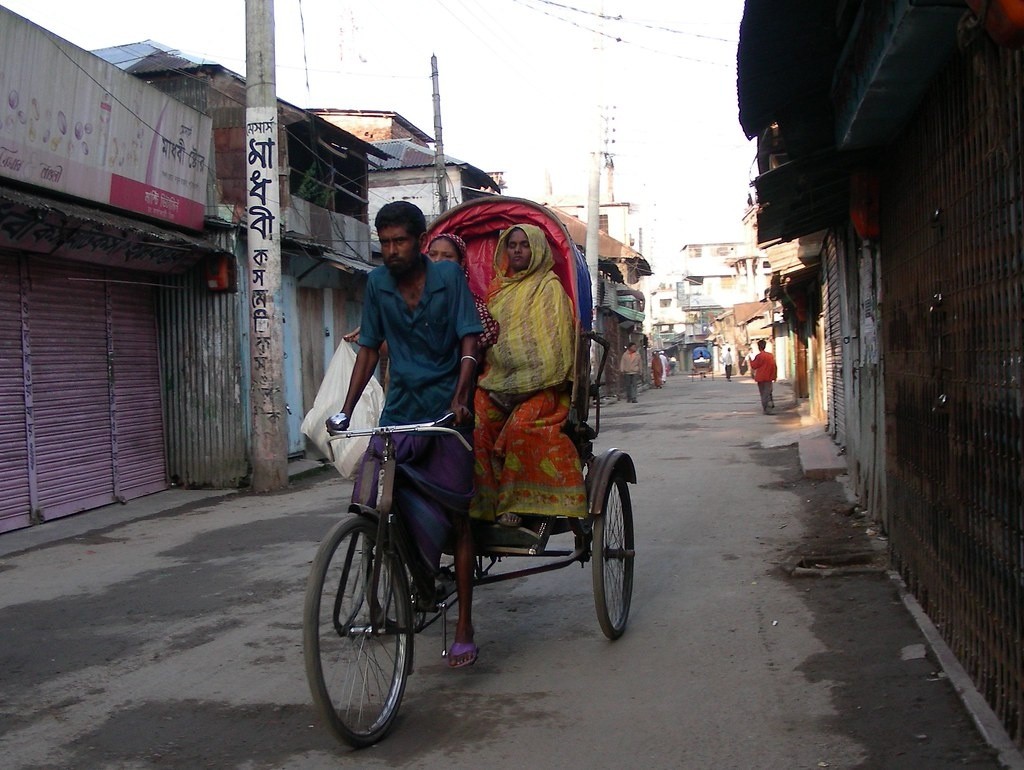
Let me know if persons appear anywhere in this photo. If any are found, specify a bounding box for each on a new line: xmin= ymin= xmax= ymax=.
xmin=343 ymin=234 xmax=500 ymax=349
xmin=670 ymin=355 xmax=676 ymax=361
xmin=659 ymin=351 xmax=668 ymax=382
xmin=651 ymin=352 xmax=663 ymax=388
xmin=749 ymin=340 xmax=777 ymax=415
xmin=724 ymin=347 xmax=732 ymax=379
xmin=326 ymin=201 xmax=483 ymax=668
xmin=698 ymin=351 xmax=706 ymax=377
xmin=471 ymin=224 xmax=588 ymax=525
xmin=742 ymin=348 xmax=756 ymax=379
xmin=621 ymin=342 xmax=642 ymax=403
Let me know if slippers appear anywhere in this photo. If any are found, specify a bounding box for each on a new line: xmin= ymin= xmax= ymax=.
xmin=447 ymin=642 xmax=476 ymax=669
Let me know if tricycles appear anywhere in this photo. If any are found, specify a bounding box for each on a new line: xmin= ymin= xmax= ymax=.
xmin=691 ymin=347 xmax=714 ymax=383
xmin=302 ymin=195 xmax=637 ymax=748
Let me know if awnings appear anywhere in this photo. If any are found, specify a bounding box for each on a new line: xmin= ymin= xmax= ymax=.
xmin=612 ymin=305 xmax=645 ymax=322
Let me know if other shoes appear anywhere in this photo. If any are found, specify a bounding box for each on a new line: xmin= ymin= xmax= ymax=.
xmin=498 ymin=513 xmax=522 ymax=527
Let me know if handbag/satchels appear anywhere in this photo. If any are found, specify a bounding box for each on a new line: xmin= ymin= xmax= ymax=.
xmin=299 ymin=338 xmax=386 ymax=483
